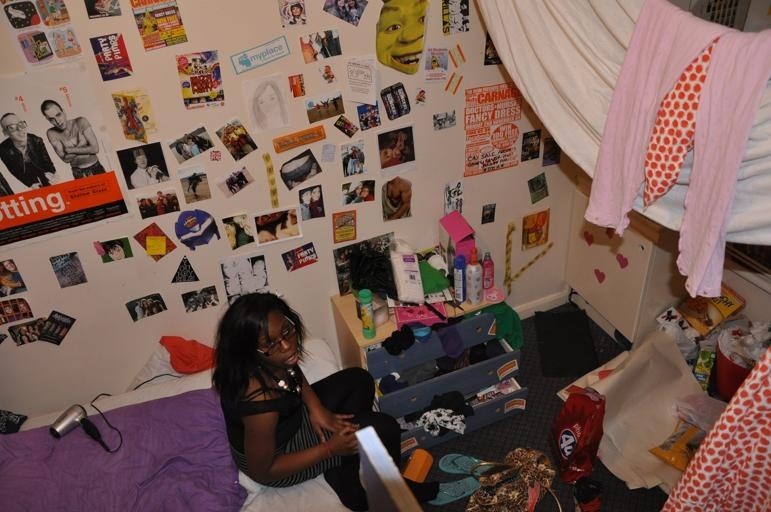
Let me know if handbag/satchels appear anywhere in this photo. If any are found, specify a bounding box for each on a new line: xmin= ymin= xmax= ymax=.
xmin=554 ymin=391 xmax=606 ymax=485
xmin=463 ymin=445 xmax=557 ymax=512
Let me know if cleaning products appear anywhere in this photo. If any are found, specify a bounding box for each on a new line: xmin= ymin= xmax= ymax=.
xmin=483 ymin=252 xmax=494 ymax=289
xmin=465 ymin=248 xmax=483 ymax=305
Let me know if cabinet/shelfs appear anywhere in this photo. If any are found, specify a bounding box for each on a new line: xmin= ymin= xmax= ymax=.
xmin=567 ymin=187 xmax=690 ymax=351
xmin=330 ymin=297 xmax=530 ymax=460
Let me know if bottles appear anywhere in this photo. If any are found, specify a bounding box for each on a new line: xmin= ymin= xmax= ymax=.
xmin=358 ymin=287 xmax=377 ymax=340
xmin=452 ymin=248 xmax=495 ymax=305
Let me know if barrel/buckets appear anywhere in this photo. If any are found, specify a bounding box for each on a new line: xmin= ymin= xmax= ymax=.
xmin=712 ymin=337 xmax=751 ymax=401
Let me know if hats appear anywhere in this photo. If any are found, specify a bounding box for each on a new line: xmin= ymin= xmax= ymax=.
xmin=347 ymin=180 xmax=363 ymax=194
xmin=133 ymin=148 xmax=146 ymax=159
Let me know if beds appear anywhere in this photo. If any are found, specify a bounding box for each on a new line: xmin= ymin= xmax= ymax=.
xmin=1 ymin=338 xmax=367 ymax=512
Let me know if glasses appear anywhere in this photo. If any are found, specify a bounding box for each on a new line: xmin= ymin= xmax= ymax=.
xmin=255 ymin=315 xmax=297 ymax=358
xmin=4 ymin=120 xmax=28 ymax=133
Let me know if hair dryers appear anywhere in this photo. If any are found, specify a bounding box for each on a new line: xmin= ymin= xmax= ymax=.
xmin=49 ymin=405 xmax=112 ymax=455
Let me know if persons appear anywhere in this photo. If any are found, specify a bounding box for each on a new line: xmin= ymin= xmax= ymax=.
xmin=0 ymin=259 xmax=29 ymax=296
xmin=40 ymin=99 xmax=107 ymax=180
xmin=133 ymin=297 xmax=165 ymax=320
xmin=341 ymin=145 xmax=365 ymax=177
xmin=135 ymin=190 xmax=179 ymax=220
xmin=220 ymin=257 xmax=267 ymax=305
xmin=102 ymin=238 xmax=126 ymax=261
xmin=173 ymin=132 xmax=212 ymax=160
xmin=361 ymin=186 xmax=376 ymax=202
xmin=224 ymin=170 xmax=248 ymax=194
xmin=342 ymin=181 xmax=365 ymax=207
xmin=446 ymin=180 xmax=464 ymax=214
xmin=229 ymin=133 xmax=258 ymax=162
xmin=117 ymin=96 xmax=138 ymax=129
xmin=311 ymin=97 xmax=339 ymax=121
xmin=207 ymin=291 xmax=439 ymax=512
xmin=375 ymin=0 xmax=428 ymax=75
xmin=415 ymin=89 xmax=427 ymax=102
xmin=331 ymin=0 xmax=353 ymax=22
xmin=310 ymin=186 xmax=326 ymax=219
xmin=187 ymin=172 xmax=201 ymax=199
xmin=430 ymin=55 xmax=441 ymax=71
xmin=286 ymin=3 xmax=308 ymax=28
xmin=232 ymin=214 xmax=254 ymax=247
xmin=322 ymin=65 xmax=336 ymax=85
xmin=1 ymin=301 xmax=68 ymax=346
xmin=185 ymin=291 xmax=217 ymax=314
xmin=222 ymin=223 xmax=237 ymax=249
xmin=251 ymin=78 xmax=289 ymax=128
xmin=381 ymin=176 xmax=413 ymax=222
xmin=129 ymin=148 xmax=164 ymax=187
xmin=346 ymin=0 xmax=363 ymax=21
xmin=0 ymin=113 xmax=59 ymax=193
xmin=299 ymin=189 xmax=313 ymax=222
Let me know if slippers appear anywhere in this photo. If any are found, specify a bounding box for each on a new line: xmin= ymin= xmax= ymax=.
xmin=427 ymin=477 xmax=482 ymax=507
xmin=438 ymin=453 xmax=495 ymax=476
